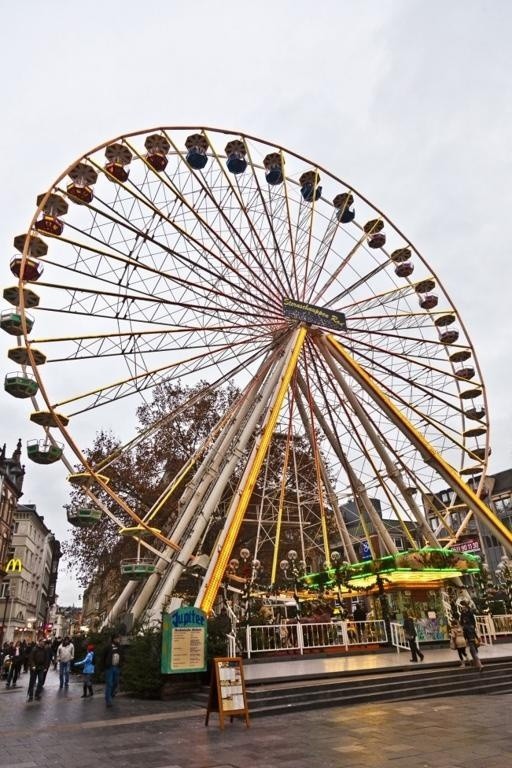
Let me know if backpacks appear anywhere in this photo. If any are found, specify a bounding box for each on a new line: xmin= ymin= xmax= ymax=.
xmin=92 ymin=657 xmax=96 ymax=664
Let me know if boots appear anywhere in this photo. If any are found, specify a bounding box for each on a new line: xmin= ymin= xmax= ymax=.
xmin=475 ymin=659 xmax=484 ymax=672
xmin=466 ymin=657 xmax=471 ymax=662
xmin=460 ymin=661 xmax=465 ymax=667
xmin=81 ymin=685 xmax=93 ymax=698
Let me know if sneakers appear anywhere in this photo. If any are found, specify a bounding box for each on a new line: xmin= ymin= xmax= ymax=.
xmin=27 ymin=696 xmax=41 ymax=702
xmin=420 ymin=654 xmax=425 ymax=661
xmin=410 ymin=658 xmax=418 ymax=662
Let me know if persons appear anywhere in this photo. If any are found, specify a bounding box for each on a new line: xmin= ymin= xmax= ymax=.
xmin=101 ymin=633 xmax=123 ymax=707
xmin=401 ymin=611 xmax=425 ymax=662
xmin=462 ymin=615 xmax=484 ymax=672
xmin=449 ymin=619 xmax=472 ymax=669
xmin=74 ymin=644 xmax=96 ymax=699
xmin=460 ymin=600 xmax=477 ymax=630
xmin=26 ymin=638 xmax=52 ymax=702
xmin=352 ymin=604 xmax=365 ymax=635
xmin=0 ymin=635 xmax=76 ymax=691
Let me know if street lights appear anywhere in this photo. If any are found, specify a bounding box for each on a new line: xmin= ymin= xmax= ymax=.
xmin=0 ymin=589 xmax=10 ymax=630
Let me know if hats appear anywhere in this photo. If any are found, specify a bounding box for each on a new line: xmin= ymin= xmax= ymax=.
xmin=87 ymin=644 xmax=95 ymax=651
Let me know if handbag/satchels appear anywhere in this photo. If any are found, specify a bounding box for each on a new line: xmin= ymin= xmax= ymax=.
xmin=405 ymin=633 xmax=414 ymax=641
xmin=449 ymin=639 xmax=456 ymax=650
xmin=474 ymin=637 xmax=482 ymax=648
